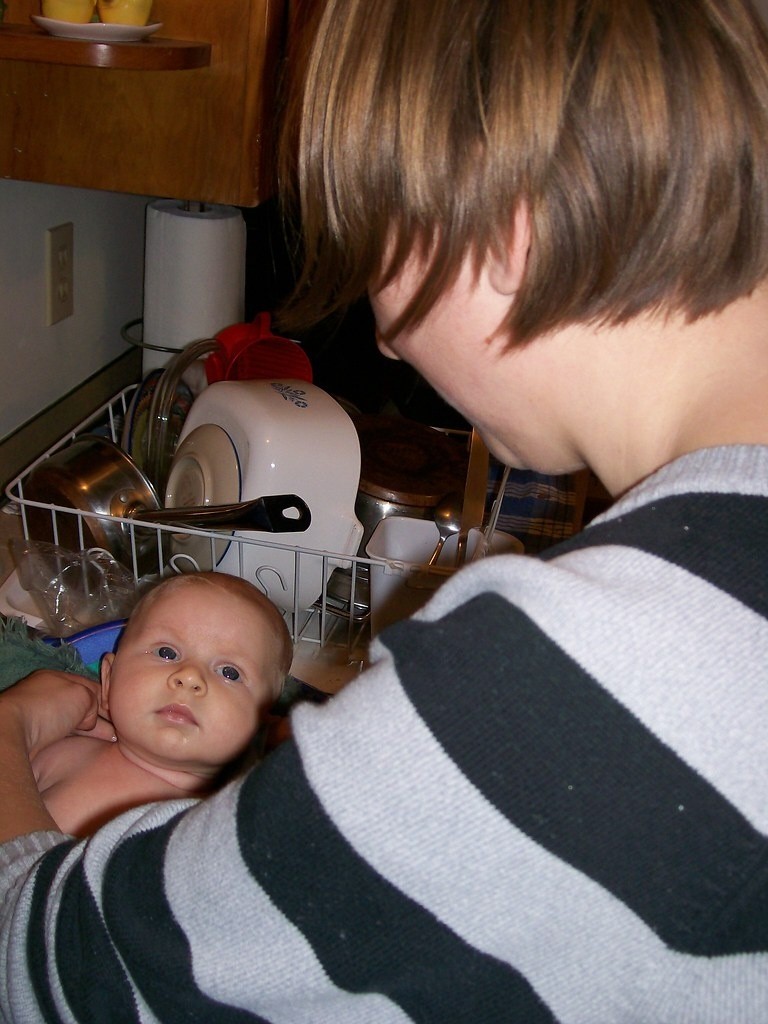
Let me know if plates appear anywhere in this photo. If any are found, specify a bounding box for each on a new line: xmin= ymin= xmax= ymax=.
xmin=120 ymin=338 xmax=218 ymax=506
xmin=162 ymin=423 xmax=242 ymax=574
xmin=30 ymin=14 xmax=164 ymax=41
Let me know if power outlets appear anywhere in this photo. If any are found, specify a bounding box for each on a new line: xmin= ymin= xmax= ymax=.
xmin=46 ymin=222 xmax=73 ymax=328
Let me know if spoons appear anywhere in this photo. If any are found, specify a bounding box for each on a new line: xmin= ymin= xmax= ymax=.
xmin=427 ymin=495 xmax=462 ymax=563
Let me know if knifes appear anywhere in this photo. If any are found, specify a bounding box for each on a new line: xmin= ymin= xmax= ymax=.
xmin=458 ymin=426 xmax=490 ymax=567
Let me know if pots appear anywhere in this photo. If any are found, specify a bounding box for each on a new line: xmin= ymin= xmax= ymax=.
xmin=24 ymin=433 xmax=312 ymax=592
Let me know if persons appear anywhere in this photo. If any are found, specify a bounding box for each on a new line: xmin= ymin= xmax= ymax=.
xmin=0 ymin=1 xmax=767 ymax=1024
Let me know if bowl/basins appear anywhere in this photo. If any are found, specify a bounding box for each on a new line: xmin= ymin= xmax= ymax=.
xmin=175 ymin=378 xmax=364 ymax=614
